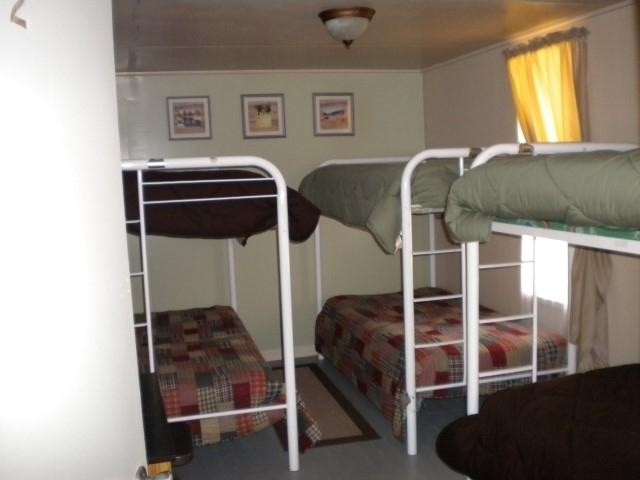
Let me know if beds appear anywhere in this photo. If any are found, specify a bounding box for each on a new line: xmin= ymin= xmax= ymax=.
xmin=436 ymin=137 xmax=640 ymax=476
xmin=121 ymin=155 xmax=323 ymax=471
xmin=298 ymin=147 xmax=542 ymax=455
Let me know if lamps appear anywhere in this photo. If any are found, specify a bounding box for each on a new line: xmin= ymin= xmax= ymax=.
xmin=317 ymin=6 xmax=377 ymax=48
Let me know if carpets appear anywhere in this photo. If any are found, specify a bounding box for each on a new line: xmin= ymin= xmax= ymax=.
xmin=270 ymin=362 xmax=382 ymax=452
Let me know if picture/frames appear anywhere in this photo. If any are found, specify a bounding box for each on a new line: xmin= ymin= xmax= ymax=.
xmin=312 ymin=91 xmax=355 ymax=137
xmin=166 ymin=93 xmax=212 ymax=141
xmin=241 ymin=92 xmax=286 ymax=140
